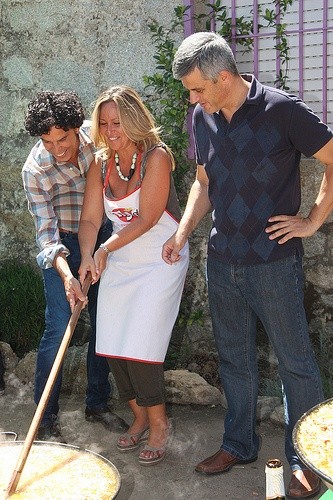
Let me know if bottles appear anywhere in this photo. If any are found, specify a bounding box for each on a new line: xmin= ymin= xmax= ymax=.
xmin=265 ymin=458 xmax=286 ymax=500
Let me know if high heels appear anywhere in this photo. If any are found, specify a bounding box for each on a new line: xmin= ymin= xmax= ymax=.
xmin=117 ymin=423 xmax=149 ymax=451
xmin=139 ymin=419 xmax=174 ymax=466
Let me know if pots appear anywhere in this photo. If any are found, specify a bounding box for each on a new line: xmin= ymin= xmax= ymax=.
xmin=0 ymin=431 xmax=122 ymax=500
xmin=292 ymin=396 xmax=333 ymax=492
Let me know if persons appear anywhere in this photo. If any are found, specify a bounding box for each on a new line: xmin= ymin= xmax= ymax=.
xmin=22 ymin=92 xmax=130 ymax=443
xmin=162 ymin=32 xmax=333 ymax=500
xmin=77 ymin=84 xmax=190 ymax=464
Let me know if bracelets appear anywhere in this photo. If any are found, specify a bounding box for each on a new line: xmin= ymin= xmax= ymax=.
xmin=100 ymin=244 xmax=111 ymax=255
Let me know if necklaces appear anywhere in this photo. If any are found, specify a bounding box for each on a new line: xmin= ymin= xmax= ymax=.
xmin=115 ymin=151 xmax=136 ymax=182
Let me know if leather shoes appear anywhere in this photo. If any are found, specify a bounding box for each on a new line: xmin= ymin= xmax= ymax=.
xmin=285 ymin=469 xmax=322 ymax=500
xmin=36 ymin=423 xmax=66 ymax=443
xmin=87 ymin=408 xmax=130 ymax=434
xmin=195 ymin=448 xmax=258 ymax=475
